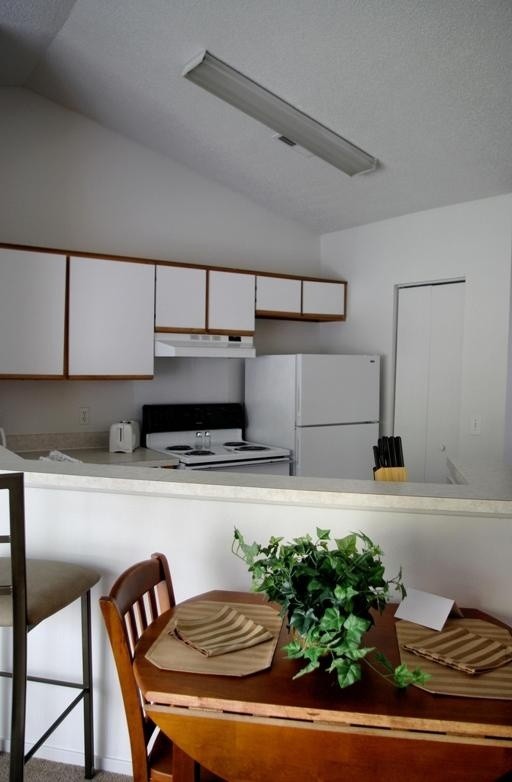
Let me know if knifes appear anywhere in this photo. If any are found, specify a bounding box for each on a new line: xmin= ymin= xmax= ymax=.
xmin=372 ymin=436 xmax=405 ymax=468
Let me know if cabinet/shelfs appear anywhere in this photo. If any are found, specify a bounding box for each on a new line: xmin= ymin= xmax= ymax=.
xmin=2 ymin=242 xmax=348 ymax=381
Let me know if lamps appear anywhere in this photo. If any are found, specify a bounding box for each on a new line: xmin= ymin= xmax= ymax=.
xmin=181 ymin=49 xmax=378 ymax=180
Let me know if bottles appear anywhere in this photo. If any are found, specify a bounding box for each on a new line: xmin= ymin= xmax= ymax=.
xmin=195 ymin=431 xmax=211 ymax=450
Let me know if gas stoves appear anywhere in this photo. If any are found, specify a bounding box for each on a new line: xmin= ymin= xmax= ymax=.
xmin=142 ymin=440 xmax=291 ymax=465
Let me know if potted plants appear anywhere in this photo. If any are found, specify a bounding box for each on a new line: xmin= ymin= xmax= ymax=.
xmin=229 ymin=524 xmax=433 ymax=690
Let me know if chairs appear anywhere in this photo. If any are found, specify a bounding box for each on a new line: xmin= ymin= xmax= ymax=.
xmin=98 ymin=551 xmax=224 ymax=780
xmin=0 ymin=471 xmax=107 ymax=780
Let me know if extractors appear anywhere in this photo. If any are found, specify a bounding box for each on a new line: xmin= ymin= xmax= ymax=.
xmin=154 ymin=332 xmax=257 ymax=359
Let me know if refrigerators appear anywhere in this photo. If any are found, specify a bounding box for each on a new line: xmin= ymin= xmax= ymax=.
xmin=244 ymin=353 xmax=380 ymax=481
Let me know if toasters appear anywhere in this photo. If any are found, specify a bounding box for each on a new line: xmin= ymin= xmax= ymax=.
xmin=109 ymin=420 xmax=140 ymax=454
